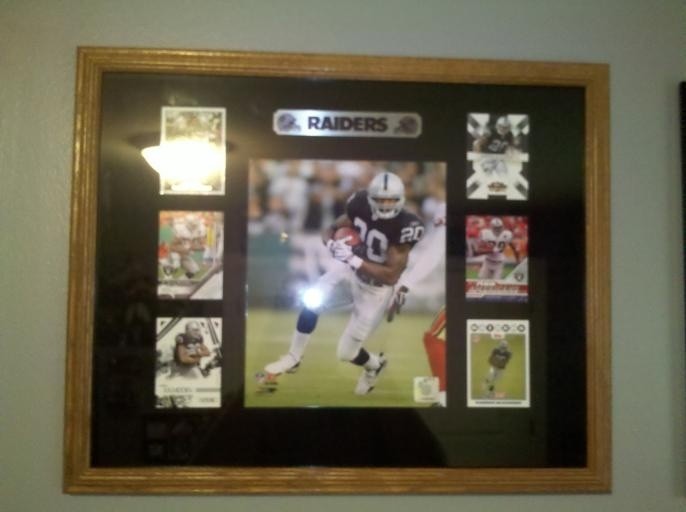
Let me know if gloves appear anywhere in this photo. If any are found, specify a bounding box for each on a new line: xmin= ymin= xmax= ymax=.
xmin=326 ymin=235 xmax=364 ymax=272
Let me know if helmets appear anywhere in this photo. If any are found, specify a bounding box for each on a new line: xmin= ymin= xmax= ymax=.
xmin=366 ymin=171 xmax=407 ymax=220
xmin=490 ymin=218 xmax=504 ymax=236
xmin=496 ymin=117 xmax=510 ymax=136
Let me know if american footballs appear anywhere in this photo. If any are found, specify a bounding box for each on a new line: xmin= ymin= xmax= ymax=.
xmin=334 ymin=226 xmax=361 ymax=247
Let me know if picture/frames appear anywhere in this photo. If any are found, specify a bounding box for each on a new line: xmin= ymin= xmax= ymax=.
xmin=62 ymin=42 xmax=617 ymax=501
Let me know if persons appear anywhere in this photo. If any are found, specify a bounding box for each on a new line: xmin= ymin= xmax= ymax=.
xmin=473 ymin=118 xmax=516 ymax=196
xmin=486 ymin=340 xmax=512 ymax=391
xmin=164 ymin=216 xmax=208 ymax=279
xmin=264 ymin=172 xmax=426 ymax=396
xmin=474 ymin=217 xmax=513 ymax=279
xmin=170 ymin=320 xmax=210 ymax=380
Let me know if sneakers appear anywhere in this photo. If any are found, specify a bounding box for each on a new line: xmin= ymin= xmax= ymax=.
xmin=355 ymin=354 xmax=385 ymax=394
xmin=264 ymin=353 xmax=300 ymax=375
